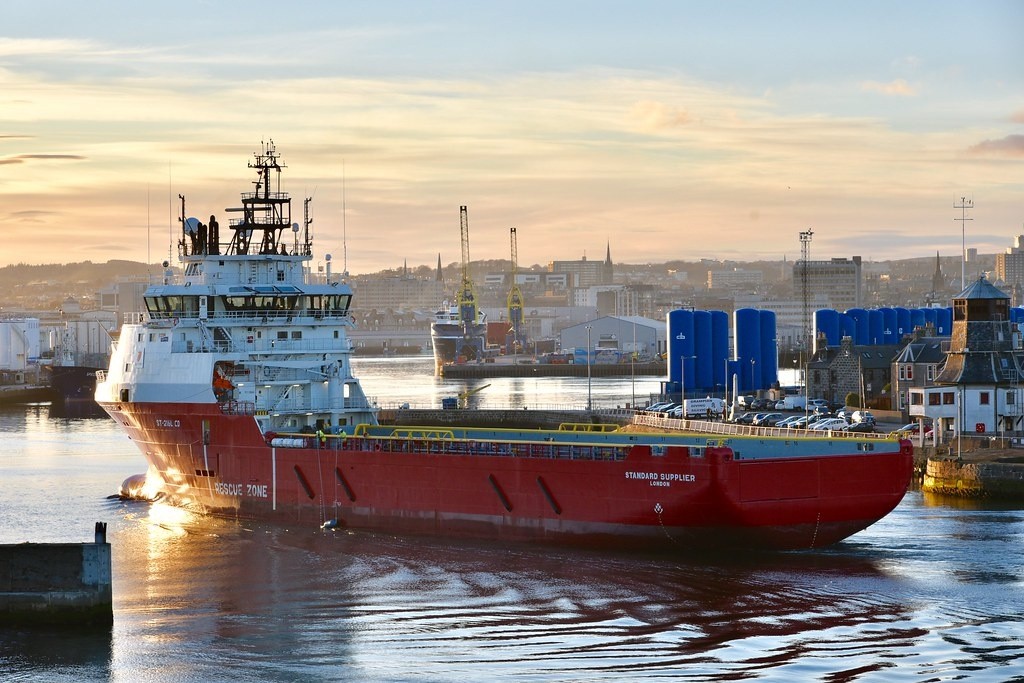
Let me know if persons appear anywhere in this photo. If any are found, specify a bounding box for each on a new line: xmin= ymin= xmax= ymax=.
xmin=715 ymin=409 xmax=718 ymax=422
xmin=316 ymin=430 xmax=326 ymax=449
xmin=722 ymin=406 xmax=726 ymax=420
xmin=337 ymin=428 xmax=348 ymax=450
xmin=707 ymin=407 xmax=712 ymax=422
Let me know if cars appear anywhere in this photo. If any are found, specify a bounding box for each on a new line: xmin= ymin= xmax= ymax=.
xmin=644 ymin=396 xmax=877 ymax=433
xmin=897 ymin=423 xmax=932 ymax=436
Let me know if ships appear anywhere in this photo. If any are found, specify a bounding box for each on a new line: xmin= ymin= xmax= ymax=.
xmin=89 ymin=132 xmax=916 ymax=574
